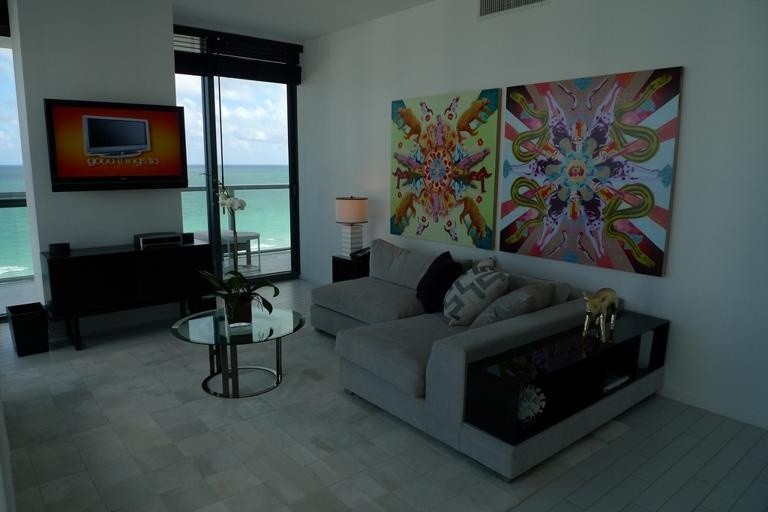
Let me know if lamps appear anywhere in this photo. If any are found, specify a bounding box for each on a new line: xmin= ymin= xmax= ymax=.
xmin=336 ymin=195 xmax=368 ymax=257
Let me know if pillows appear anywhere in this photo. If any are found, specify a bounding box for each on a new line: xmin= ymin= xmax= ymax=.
xmin=415 ymin=250 xmax=555 ymax=327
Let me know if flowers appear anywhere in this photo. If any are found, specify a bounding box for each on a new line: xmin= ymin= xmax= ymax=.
xmin=197 ymin=172 xmax=280 ymax=315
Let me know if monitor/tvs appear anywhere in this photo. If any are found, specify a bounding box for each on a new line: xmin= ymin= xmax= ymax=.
xmin=82 ymin=114 xmax=151 ymax=159
xmin=43 ymin=97 xmax=189 ymax=192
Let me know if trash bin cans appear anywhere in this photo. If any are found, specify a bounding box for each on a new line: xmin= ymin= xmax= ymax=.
xmin=6 ymin=302 xmax=48 ymax=357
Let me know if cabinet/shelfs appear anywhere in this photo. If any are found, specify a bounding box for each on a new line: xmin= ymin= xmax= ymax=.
xmin=40 ymin=238 xmax=214 ymax=351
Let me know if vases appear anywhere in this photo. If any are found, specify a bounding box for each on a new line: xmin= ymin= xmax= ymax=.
xmin=228 ymin=299 xmax=251 ymax=324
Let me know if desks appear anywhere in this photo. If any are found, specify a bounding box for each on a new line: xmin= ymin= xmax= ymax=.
xmin=333 ymin=255 xmax=369 ymax=283
xmin=194 ymin=232 xmax=261 ymax=276
